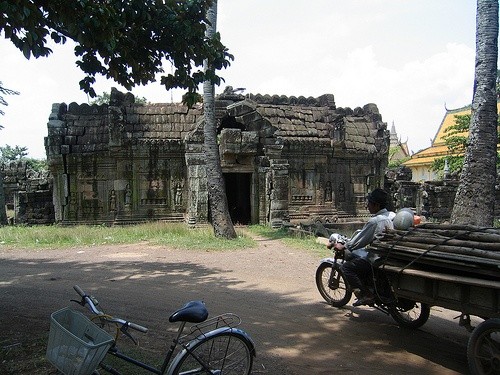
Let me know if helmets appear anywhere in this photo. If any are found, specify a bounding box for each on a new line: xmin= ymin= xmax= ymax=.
xmin=393 ymin=208 xmax=414 ymax=229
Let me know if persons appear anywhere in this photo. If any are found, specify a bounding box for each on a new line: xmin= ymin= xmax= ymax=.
xmin=125 ymin=184 xmax=131 ymax=205
xmin=109 ymin=189 xmax=117 ymax=211
xmin=333 ymin=188 xmax=397 ymax=306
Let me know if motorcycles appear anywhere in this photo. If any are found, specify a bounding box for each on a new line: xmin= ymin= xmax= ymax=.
xmin=316 ymin=222 xmax=500 ymax=375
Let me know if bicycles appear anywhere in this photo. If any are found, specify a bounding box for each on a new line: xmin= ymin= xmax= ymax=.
xmin=44 ymin=284 xmax=257 ymax=375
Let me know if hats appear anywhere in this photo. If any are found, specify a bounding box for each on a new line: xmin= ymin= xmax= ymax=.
xmin=365 ymin=188 xmax=391 ymax=206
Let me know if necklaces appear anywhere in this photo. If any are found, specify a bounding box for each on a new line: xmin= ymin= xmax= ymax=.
xmin=175 ymin=182 xmax=181 ymax=205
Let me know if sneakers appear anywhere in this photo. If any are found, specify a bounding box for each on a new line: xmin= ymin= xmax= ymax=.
xmin=353 ymin=296 xmax=375 ymax=307
